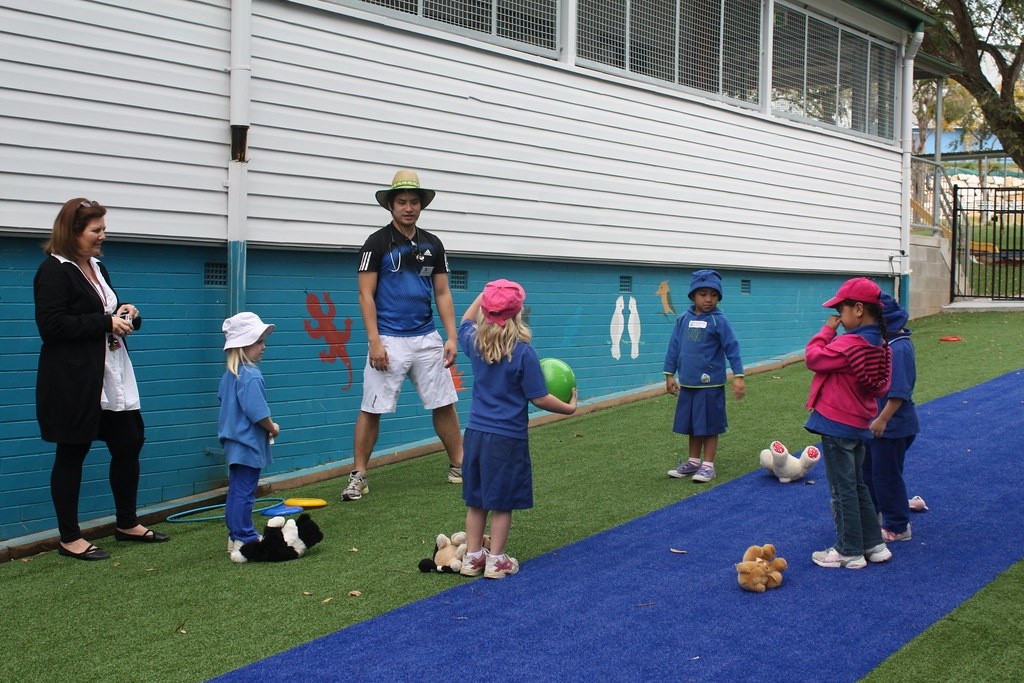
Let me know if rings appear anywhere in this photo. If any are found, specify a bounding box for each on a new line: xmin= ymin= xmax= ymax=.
xmin=119 ymin=330 xmax=124 ymax=335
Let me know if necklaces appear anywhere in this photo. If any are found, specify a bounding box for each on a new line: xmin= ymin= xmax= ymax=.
xmin=76 ymin=261 xmax=121 ymax=351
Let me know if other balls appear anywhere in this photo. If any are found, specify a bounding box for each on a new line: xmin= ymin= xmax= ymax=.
xmin=539 ymin=358 xmax=576 ymax=403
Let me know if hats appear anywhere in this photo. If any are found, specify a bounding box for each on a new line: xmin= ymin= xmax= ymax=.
xmin=374 ymin=170 xmax=436 ymax=211
xmin=222 ymin=311 xmax=275 ymax=351
xmin=688 ymin=270 xmax=722 ymax=302
xmin=822 ymin=277 xmax=884 ymax=308
xmin=479 ymin=279 xmax=526 ymax=326
xmin=881 ymin=294 xmax=909 ymax=333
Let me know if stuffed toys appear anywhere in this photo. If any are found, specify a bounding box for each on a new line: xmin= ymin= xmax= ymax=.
xmin=239 ymin=513 xmax=324 ymax=563
xmin=418 ymin=531 xmax=493 ymax=574
xmin=759 ymin=441 xmax=820 ymax=484
xmin=908 ymin=496 xmax=928 ymax=511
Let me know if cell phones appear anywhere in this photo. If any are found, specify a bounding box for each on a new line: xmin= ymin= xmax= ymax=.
xmin=119 ymin=315 xmax=141 ymax=330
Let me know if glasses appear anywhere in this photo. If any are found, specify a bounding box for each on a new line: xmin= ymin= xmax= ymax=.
xmin=77 ymin=201 xmax=99 ymax=211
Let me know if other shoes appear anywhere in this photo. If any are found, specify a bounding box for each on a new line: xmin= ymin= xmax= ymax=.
xmin=115 ymin=529 xmax=169 ymax=542
xmin=59 ymin=542 xmax=110 ymax=561
xmin=228 ymin=534 xmax=263 ymax=555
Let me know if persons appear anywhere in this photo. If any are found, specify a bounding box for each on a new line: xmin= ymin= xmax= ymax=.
xmin=663 ymin=270 xmax=746 ymax=482
xmin=805 ymin=277 xmax=892 ymax=569
xmin=458 ymin=279 xmax=577 ymax=579
xmin=871 ymin=294 xmax=920 ymax=542
xmin=217 ymin=312 xmax=279 ymax=553
xmin=341 ymin=171 xmax=466 ymax=501
xmin=33 ymin=198 xmax=169 ymax=561
xmin=736 ymin=544 xmax=788 ymax=592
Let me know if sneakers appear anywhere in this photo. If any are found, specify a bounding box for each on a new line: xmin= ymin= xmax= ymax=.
xmin=881 ymin=523 xmax=912 ymax=542
xmin=877 ymin=512 xmax=882 ymax=526
xmin=448 ymin=464 xmax=463 ymax=483
xmin=811 ymin=547 xmax=867 ymax=569
xmin=341 ymin=470 xmax=369 ymax=501
xmin=484 ymin=554 xmax=519 ymax=579
xmin=863 ymin=543 xmax=892 ymax=562
xmin=691 ymin=466 xmax=716 ymax=483
xmin=667 ymin=460 xmax=701 ymax=478
xmin=460 ymin=547 xmax=490 ymax=576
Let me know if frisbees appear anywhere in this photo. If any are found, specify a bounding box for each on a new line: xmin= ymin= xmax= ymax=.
xmin=261 ymin=505 xmax=304 ymax=516
xmin=284 ymin=498 xmax=327 ymax=507
